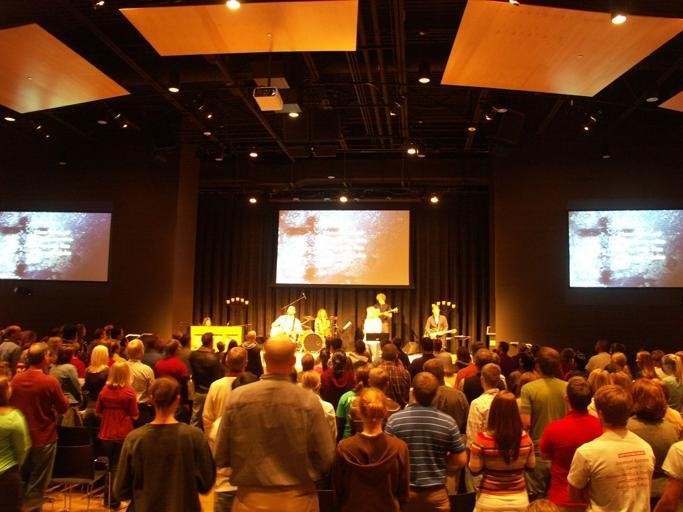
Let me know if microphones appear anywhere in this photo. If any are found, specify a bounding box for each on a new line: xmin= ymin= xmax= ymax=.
xmin=303 ymin=292 xmax=307 ymax=299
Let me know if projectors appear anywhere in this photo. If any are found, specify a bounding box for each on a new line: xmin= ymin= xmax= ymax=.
xmin=253 ymin=87 xmax=283 ymax=111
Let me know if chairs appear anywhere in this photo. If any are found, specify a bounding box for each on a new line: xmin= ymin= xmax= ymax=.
xmin=51 ymin=426 xmax=111 ymax=512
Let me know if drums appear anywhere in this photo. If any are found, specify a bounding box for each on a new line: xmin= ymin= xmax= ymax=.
xmin=302 ymin=333 xmax=324 ymax=352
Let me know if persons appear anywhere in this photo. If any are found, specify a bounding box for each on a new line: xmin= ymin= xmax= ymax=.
xmin=202 ymin=293 xmax=448 ymax=347
xmin=0 ymin=324 xmax=682 ymax=512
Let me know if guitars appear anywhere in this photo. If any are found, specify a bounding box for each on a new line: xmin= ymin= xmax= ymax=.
xmin=422 ymin=329 xmax=457 ymax=341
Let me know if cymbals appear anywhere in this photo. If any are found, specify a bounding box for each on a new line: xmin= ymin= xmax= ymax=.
xmin=301 ymin=316 xmax=314 ymax=320
xmin=326 ymin=316 xmax=339 ymax=320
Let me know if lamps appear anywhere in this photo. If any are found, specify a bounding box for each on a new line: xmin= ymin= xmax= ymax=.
xmin=611 ymin=8 xmax=627 ymax=25
xmin=418 ymin=62 xmax=431 ymax=83
